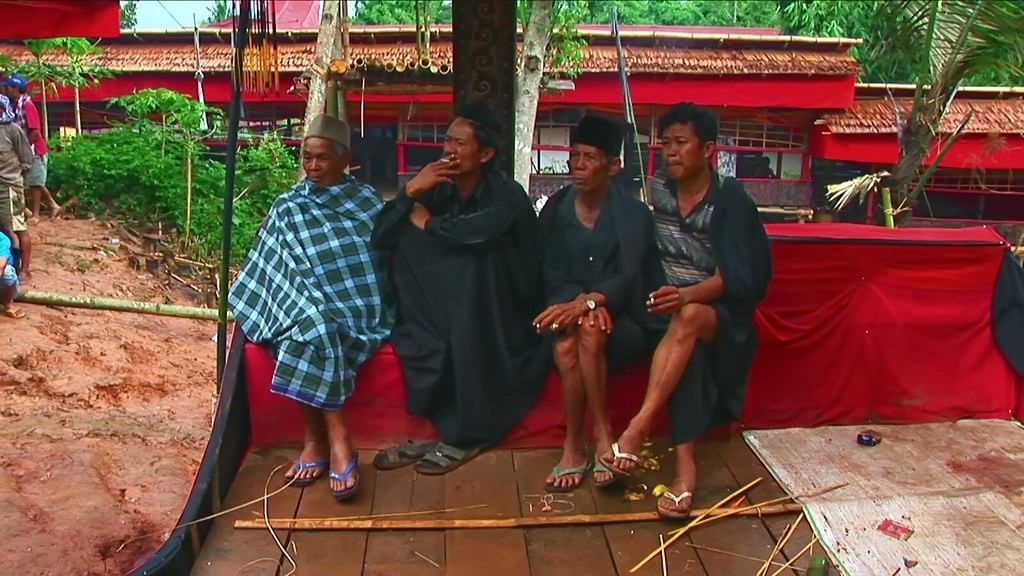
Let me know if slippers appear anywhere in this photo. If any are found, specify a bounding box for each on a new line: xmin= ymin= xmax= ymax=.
xmin=598 ymin=442 xmax=643 ymax=477
xmin=543 ymin=455 xmax=592 ymax=493
xmin=285 ymin=460 xmax=326 ymax=483
xmin=328 ymin=451 xmax=360 ymax=499
xmin=592 ymin=467 xmax=619 ymax=487
xmin=656 ymin=490 xmax=693 ymax=519
xmin=413 ymin=441 xmax=481 ymax=474
xmin=372 ymin=439 xmax=439 ymax=469
xmin=5 ymin=307 xmax=25 ymax=317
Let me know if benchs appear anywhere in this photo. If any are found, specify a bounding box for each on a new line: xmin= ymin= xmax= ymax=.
xmin=247 ymin=341 xmax=742 ymax=455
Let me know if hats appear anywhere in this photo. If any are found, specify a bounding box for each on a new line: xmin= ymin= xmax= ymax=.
xmin=305 ymin=114 xmax=349 ymax=151
xmin=571 ymin=112 xmax=627 ymax=156
xmin=0 ymin=73 xmax=26 ymax=88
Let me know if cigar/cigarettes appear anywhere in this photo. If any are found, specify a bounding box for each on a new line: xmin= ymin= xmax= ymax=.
xmin=441 ymin=159 xmax=450 ymax=163
xmin=651 ymin=298 xmax=654 ymax=304
xmin=537 ymin=323 xmax=540 ymax=328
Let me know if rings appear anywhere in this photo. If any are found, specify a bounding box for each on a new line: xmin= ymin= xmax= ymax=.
xmin=552 ymin=323 xmax=559 ymax=328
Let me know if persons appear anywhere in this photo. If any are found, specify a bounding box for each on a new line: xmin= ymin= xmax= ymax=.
xmin=371 ymin=110 xmax=537 ymax=447
xmin=0 ymin=73 xmax=61 ymax=317
xmin=532 ymin=113 xmax=652 ymax=492
xmin=227 ymin=114 xmax=390 ymax=499
xmin=600 ymin=101 xmax=772 ymax=519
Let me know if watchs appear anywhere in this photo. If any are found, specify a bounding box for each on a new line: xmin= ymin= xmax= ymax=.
xmin=583 ymin=295 xmax=596 ymax=310
